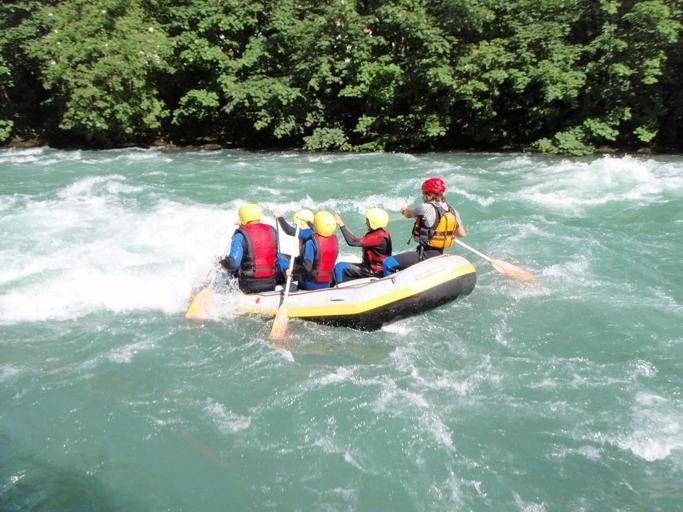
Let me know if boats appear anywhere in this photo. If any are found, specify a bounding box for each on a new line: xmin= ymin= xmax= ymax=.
xmin=184 ymin=247 xmax=476 ymax=332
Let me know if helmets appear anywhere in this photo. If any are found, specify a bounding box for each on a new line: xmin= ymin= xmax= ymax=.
xmin=238 ymin=203 xmax=261 ymax=225
xmin=364 ymin=207 xmax=389 ymax=231
xmin=313 ymin=210 xmax=337 ymax=238
xmin=421 ymin=178 xmax=446 ymax=193
xmin=292 ymin=209 xmax=315 ymax=229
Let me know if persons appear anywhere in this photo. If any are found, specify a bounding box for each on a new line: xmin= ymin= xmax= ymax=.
xmin=223 ymin=203 xmax=280 ymax=292
xmin=331 ymin=208 xmax=391 ymax=286
xmin=281 ymin=211 xmax=339 ymax=293
xmin=391 ymin=177 xmax=465 ymax=270
xmin=270 ymin=206 xmax=314 ymax=282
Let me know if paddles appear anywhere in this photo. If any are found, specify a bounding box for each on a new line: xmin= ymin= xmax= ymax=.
xmin=414 ymin=216 xmax=521 ymax=274
xmin=269 ymin=218 xmax=302 ymax=332
xmin=185 ymin=222 xmax=240 ymax=316
